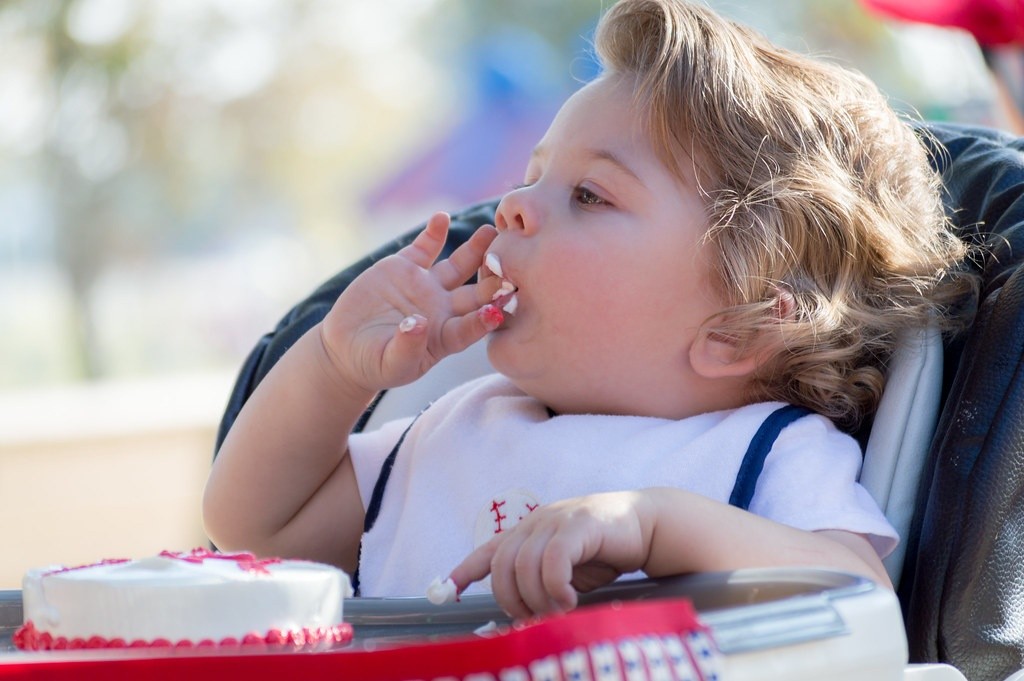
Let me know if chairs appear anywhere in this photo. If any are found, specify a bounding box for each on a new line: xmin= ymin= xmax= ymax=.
xmin=1 ymin=111 xmax=1024 ymax=681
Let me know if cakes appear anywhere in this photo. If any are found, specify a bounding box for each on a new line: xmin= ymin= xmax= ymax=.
xmin=12 ymin=547 xmax=355 ymax=653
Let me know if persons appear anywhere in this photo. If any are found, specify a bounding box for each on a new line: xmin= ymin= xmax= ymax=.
xmin=200 ymin=0 xmax=978 ymax=619
